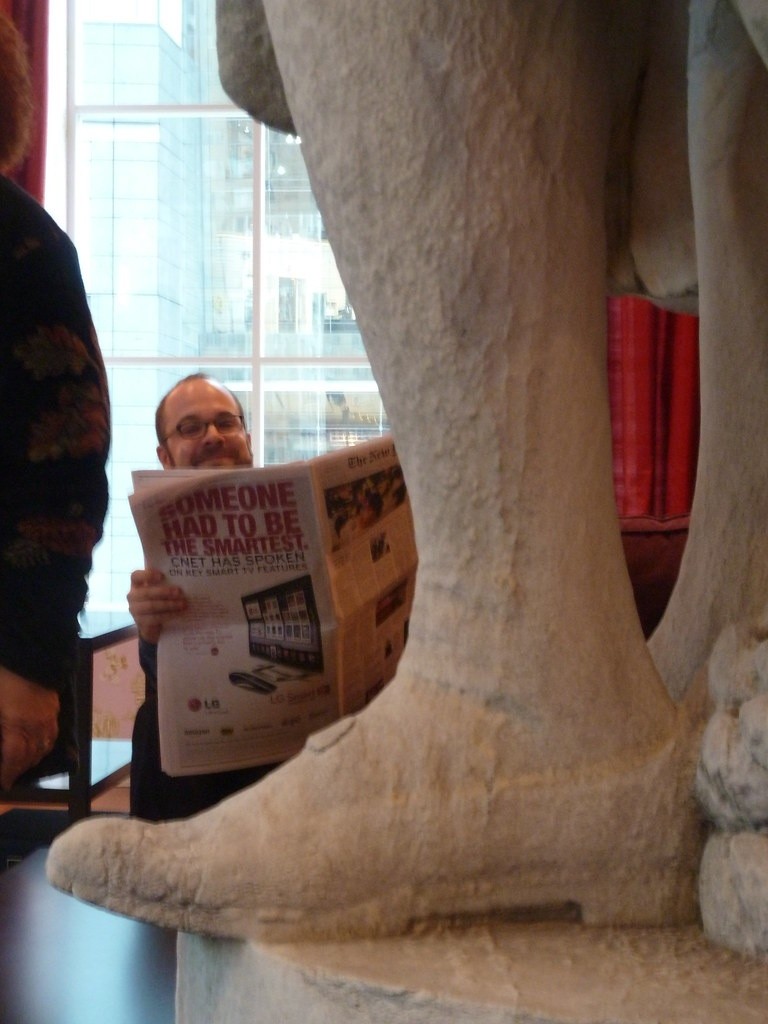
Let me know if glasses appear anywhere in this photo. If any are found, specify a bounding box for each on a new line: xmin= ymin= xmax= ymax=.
xmin=163 ymin=414 xmax=244 ymax=444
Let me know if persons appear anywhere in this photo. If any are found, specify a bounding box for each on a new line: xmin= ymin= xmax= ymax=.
xmin=0 ymin=7 xmax=112 ymax=873
xmin=52 ymin=0 xmax=767 ymax=946
xmin=131 ymin=372 xmax=286 ymax=826
xmin=328 ymin=465 xmax=407 ymax=547
